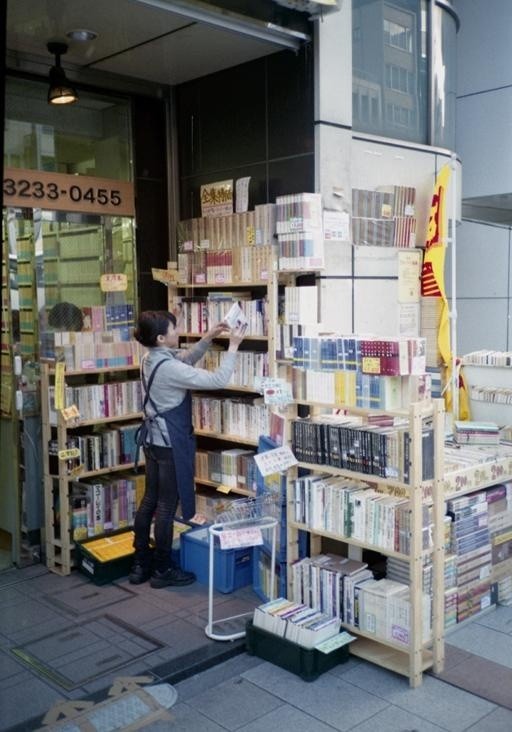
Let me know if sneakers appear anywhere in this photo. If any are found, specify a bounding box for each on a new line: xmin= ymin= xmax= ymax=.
xmin=151 ymin=560 xmax=196 ymax=588
xmin=128 ymin=561 xmax=152 ymax=584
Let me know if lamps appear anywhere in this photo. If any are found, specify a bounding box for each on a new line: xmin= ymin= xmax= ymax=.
xmin=45 ymin=42 xmax=79 ymax=105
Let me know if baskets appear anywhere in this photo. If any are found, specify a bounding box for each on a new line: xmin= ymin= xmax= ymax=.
xmin=216 ymin=493 xmax=276 ymax=530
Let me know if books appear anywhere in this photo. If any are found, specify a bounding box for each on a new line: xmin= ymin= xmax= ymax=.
xmin=42 ymin=209 xmax=138 ymax=306
xmin=42 ymin=306 xmax=157 ymax=563
xmin=173 ymin=193 xmax=511 ymax=651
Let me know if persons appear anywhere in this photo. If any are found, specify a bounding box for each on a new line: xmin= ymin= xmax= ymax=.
xmin=47 ymin=302 xmax=100 ymax=555
xmin=128 ymin=312 xmax=248 ymax=588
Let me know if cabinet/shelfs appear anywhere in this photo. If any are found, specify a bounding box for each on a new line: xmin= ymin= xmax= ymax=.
xmin=0 ymin=217 xmax=512 ymax=690
